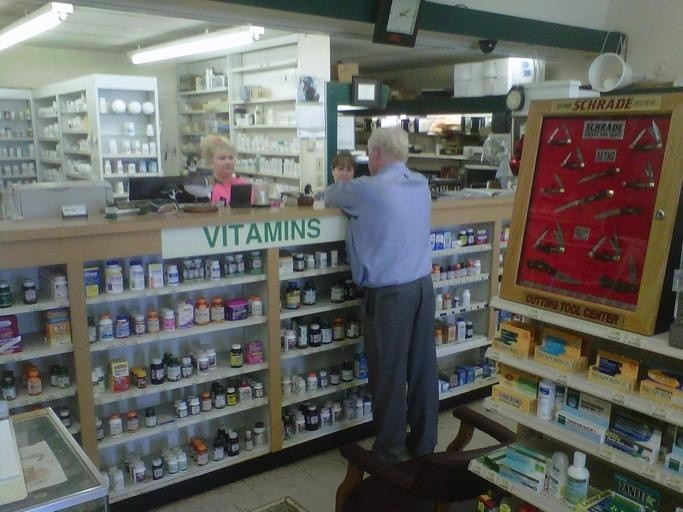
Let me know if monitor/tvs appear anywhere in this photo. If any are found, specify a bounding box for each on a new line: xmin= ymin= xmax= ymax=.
xmin=129 ymin=175 xmax=192 ymax=202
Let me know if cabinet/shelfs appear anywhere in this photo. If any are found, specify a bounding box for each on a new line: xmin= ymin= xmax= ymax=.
xmin=0 ymin=31 xmax=681 ymax=511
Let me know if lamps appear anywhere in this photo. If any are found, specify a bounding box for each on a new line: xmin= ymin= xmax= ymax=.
xmin=1 ymin=2 xmax=73 ymax=53
xmin=127 ymin=25 xmax=265 ymax=66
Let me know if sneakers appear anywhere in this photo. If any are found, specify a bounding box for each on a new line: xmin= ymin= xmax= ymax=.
xmin=405 ymin=431 xmax=435 ymax=458
xmin=371 ymin=439 xmax=412 ymax=463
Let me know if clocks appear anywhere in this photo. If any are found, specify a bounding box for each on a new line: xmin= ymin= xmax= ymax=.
xmin=372 ymin=0 xmax=426 ymax=47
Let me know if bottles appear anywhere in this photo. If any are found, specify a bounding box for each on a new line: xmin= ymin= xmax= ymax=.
xmin=279 ymin=248 xmax=380 ymax=436
xmin=547 ymin=450 xmax=590 ymax=507
xmin=430 ymin=222 xmax=493 ymax=346
xmin=534 ymin=375 xmax=566 ymax=423
xmin=116 ymin=180 xmax=125 ymax=195
xmin=0 ymin=250 xmax=269 ymax=496
xmin=105 ymin=139 xmax=157 ymax=176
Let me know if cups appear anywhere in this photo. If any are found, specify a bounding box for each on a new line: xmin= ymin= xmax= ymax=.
xmin=588 ymin=51 xmax=633 ymax=93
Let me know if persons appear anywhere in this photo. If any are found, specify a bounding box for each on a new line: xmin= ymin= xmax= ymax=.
xmin=193 ymin=133 xmax=253 ymax=206
xmin=331 ymin=150 xmax=357 ymax=184
xmin=325 ymin=126 xmax=438 ymax=491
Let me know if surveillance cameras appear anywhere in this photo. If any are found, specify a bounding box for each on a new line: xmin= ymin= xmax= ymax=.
xmin=479 ymin=40 xmax=496 ymax=53
